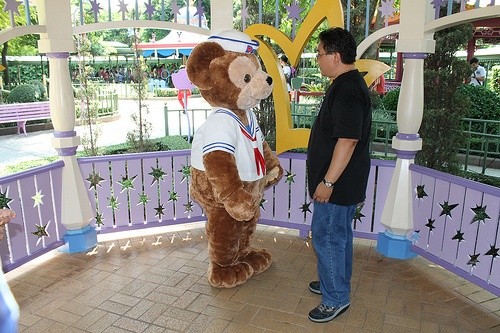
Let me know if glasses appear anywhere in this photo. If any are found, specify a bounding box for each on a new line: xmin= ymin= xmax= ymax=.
xmin=314 ymin=49 xmax=338 ymax=60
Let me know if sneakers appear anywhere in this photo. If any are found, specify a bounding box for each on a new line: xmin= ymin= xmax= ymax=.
xmin=308 ymin=302 xmax=350 ymax=323
xmin=309 ymin=282 xmax=323 ymax=294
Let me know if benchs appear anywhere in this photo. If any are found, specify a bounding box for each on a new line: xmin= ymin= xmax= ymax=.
xmin=0 ymin=101 xmax=50 ymax=137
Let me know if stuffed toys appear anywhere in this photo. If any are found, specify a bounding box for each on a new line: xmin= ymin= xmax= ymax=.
xmin=171 ymin=67 xmax=194 ymax=113
xmin=187 ymin=30 xmax=282 ymax=290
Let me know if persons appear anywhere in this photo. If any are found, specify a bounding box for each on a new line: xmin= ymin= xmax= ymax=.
xmin=0 ymin=208 xmax=19 ymax=333
xmin=69 ymin=58 xmax=184 ymax=89
xmin=470 ymin=58 xmax=487 ymax=87
xmin=280 ymin=55 xmax=291 ymax=93
xmin=307 ymin=27 xmax=371 ymax=322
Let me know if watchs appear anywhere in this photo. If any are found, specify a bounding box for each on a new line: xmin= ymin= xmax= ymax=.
xmin=322 ymin=178 xmax=334 ymax=187
